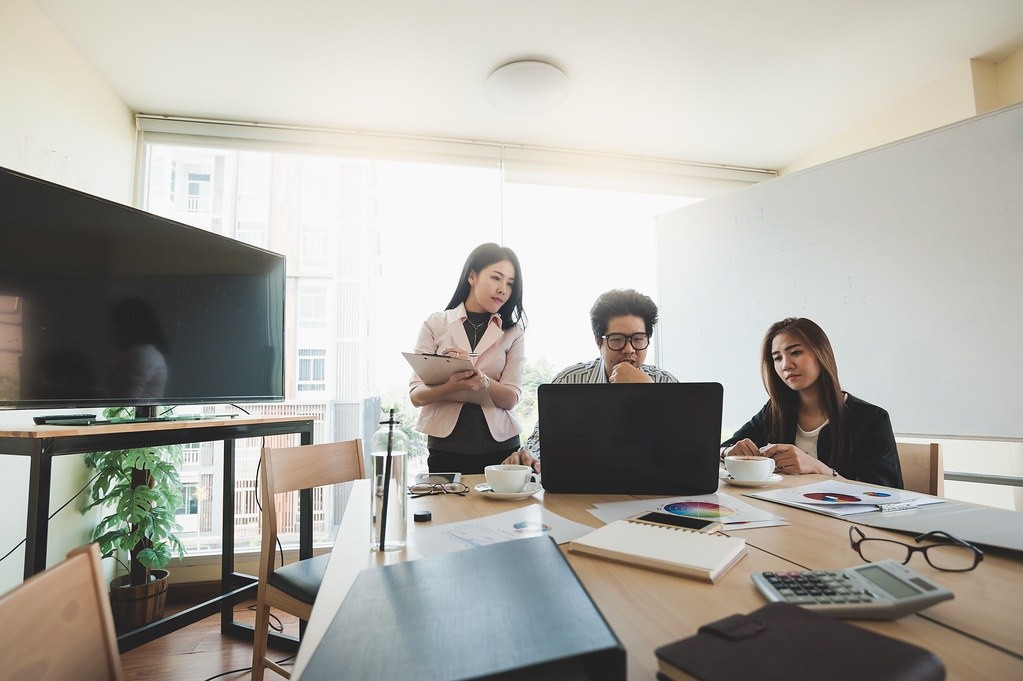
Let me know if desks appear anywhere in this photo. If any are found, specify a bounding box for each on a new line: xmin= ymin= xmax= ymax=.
xmin=289 ymin=464 xmax=1023 ymax=681
xmin=0 ymin=413 xmax=318 ymax=656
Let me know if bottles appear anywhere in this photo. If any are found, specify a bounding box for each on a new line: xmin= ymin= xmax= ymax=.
xmin=370 ymin=413 xmax=410 ymax=552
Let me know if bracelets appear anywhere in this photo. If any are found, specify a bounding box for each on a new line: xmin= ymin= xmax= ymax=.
xmin=830 ymin=467 xmax=838 ymax=477
xmin=481 ymin=372 xmax=490 ymax=392
xmin=721 ymin=446 xmax=729 ymax=460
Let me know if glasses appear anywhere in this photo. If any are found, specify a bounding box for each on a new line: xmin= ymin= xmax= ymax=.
xmin=848 ymin=524 xmax=983 ymax=572
xmin=601 ymin=331 xmax=650 ymax=352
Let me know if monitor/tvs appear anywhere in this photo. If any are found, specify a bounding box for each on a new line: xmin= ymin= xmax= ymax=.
xmin=0 ymin=166 xmax=286 ymax=423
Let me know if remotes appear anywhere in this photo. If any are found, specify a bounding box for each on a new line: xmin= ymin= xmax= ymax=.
xmin=33 ymin=414 xmax=97 ymax=425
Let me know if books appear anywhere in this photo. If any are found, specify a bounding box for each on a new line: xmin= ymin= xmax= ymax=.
xmin=653 ymin=601 xmax=945 ymax=681
xmin=568 ymin=520 xmax=748 ymax=585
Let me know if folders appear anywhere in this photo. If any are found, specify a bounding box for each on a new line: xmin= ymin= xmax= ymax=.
xmin=298 ymin=534 xmax=628 ymax=681
xmin=740 ymin=477 xmax=1023 ymax=552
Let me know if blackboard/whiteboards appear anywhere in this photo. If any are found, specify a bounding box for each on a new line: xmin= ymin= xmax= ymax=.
xmin=654 ymin=102 xmax=1023 ymax=443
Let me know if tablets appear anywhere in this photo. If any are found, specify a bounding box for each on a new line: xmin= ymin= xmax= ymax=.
xmin=408 ymin=472 xmax=462 ymax=490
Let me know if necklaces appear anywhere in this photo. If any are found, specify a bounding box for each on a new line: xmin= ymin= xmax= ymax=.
xmin=467 ymin=316 xmax=490 ymax=348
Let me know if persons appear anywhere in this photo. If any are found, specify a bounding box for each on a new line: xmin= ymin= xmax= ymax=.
xmin=409 ymin=242 xmax=529 ymax=475
xmin=501 ymin=287 xmax=681 ymax=474
xmin=720 ymin=316 xmax=904 ymax=490
xmin=112 ymin=298 xmax=174 ymax=403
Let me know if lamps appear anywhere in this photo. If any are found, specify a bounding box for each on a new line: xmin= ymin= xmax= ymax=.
xmin=479 ymin=60 xmax=574 ymax=118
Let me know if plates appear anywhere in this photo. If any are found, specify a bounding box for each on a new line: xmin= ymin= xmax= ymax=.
xmin=474 ymin=482 xmax=542 ymax=501
xmin=718 ymin=469 xmax=783 ymax=487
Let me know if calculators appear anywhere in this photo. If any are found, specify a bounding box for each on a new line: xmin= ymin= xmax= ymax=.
xmin=752 ymin=559 xmax=955 ymax=620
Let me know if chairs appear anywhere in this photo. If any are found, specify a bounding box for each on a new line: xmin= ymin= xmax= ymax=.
xmin=248 ymin=440 xmax=367 ymax=681
xmin=892 ymin=441 xmax=947 ymax=497
xmin=0 ymin=538 xmax=125 ymax=681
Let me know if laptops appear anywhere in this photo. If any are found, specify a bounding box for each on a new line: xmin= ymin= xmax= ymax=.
xmin=537 ymin=382 xmax=723 ymax=496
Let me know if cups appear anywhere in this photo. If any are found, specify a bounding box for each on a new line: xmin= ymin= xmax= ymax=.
xmin=484 ymin=464 xmax=539 ymax=493
xmin=724 ymin=455 xmax=775 ymax=481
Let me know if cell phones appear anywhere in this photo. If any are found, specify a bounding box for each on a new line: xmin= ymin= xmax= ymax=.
xmin=627 ymin=509 xmax=723 ymax=534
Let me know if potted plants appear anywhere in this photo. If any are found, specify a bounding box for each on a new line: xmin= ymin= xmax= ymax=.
xmin=77 ymin=399 xmax=186 ymax=631
